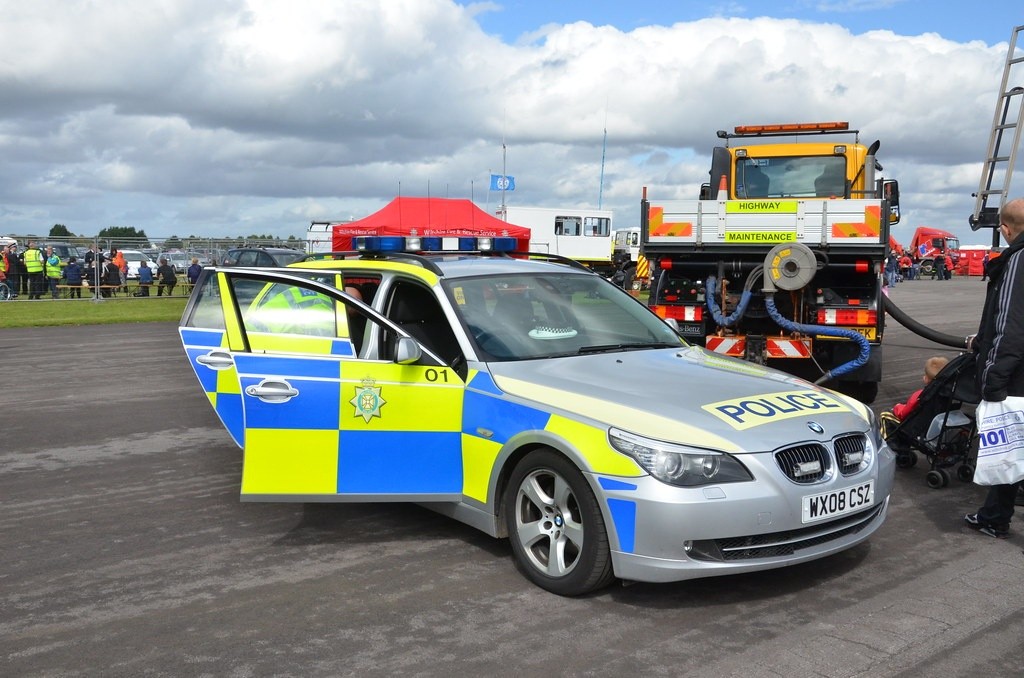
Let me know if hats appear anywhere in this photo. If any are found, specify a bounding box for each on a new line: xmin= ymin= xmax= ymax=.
xmin=528 ymin=321 xmax=579 ymax=340
xmin=8 ymin=243 xmax=17 ymax=248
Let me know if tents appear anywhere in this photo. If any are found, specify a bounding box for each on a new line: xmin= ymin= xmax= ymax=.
xmin=332 ymin=197 xmax=531 ymax=260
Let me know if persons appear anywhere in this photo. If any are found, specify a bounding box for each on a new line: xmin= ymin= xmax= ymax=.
xmin=138 ymin=261 xmax=153 ymax=296
xmin=154 ymin=259 xmax=177 ymax=296
xmin=0 ymin=243 xmax=61 ymax=299
xmin=886 ymin=250 xmax=920 ymax=287
xmin=188 ymin=258 xmax=202 ymax=288
xmin=932 ymin=255 xmax=954 ymax=280
xmin=85 ymin=245 xmax=129 ymax=298
xmin=981 ymin=250 xmax=989 ymax=280
xmin=243 ymin=286 xmax=363 ymax=336
xmin=63 ymin=258 xmax=82 ymax=298
xmin=879 ymin=357 xmax=950 ymax=439
xmin=963 ymin=199 xmax=1024 ymax=535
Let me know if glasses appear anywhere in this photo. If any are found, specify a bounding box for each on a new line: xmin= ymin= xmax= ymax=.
xmin=997 ymin=223 xmax=1008 ymax=233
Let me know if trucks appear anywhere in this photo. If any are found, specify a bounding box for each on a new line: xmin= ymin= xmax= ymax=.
xmin=496 ymin=204 xmax=652 ymax=293
xmin=889 ymin=227 xmax=962 ymax=278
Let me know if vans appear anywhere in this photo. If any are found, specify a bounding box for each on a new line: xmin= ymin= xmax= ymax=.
xmin=0 ymin=236 xmax=20 ymax=255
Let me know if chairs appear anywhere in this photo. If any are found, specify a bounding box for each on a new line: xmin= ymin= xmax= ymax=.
xmin=814 ymin=160 xmax=846 ymax=198
xmin=736 ymin=165 xmax=769 ymax=198
xmin=345 ymin=290 xmax=556 ymax=367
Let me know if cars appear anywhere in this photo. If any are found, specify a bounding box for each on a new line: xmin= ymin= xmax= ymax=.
xmin=103 ymin=249 xmax=159 ymax=279
xmin=186 ymin=252 xmax=212 ymax=268
xmin=177 ymin=229 xmax=899 ymax=600
xmin=156 ymin=253 xmax=193 ymax=274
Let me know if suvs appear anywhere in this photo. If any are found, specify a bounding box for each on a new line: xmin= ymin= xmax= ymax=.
xmin=38 ymin=242 xmax=91 ymax=276
xmin=214 ymin=244 xmax=315 ymax=299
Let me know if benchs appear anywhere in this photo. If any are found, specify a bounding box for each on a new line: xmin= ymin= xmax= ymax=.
xmin=56 ymin=285 xmax=120 ymax=298
xmin=119 ymin=284 xmax=173 ymax=296
xmin=181 ymin=284 xmax=213 ymax=296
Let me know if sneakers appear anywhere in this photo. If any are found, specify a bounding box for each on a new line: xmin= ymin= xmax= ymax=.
xmin=965 ymin=511 xmax=1007 ymax=538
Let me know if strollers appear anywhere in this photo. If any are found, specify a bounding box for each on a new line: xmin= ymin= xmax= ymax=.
xmin=883 ymin=348 xmax=982 ymax=490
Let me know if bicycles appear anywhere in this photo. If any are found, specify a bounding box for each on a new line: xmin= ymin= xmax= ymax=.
xmin=0 ymin=282 xmax=11 ymax=302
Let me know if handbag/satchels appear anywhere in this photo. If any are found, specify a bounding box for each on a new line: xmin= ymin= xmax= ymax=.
xmin=972 ymin=397 xmax=1024 ymax=485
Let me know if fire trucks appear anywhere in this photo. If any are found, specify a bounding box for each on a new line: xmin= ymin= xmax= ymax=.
xmin=635 ymin=122 xmax=901 ymax=409
